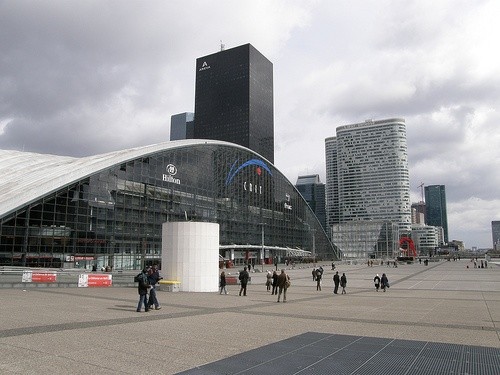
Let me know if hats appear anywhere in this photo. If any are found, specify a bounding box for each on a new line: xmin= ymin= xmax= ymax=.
xmin=317 ymin=272 xmax=321 ymax=275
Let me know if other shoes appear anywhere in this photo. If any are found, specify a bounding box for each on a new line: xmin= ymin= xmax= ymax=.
xmin=145 ymin=310 xmax=150 ymax=312
xmin=275 ymin=293 xmax=277 ymax=295
xmin=283 ymin=300 xmax=287 ymax=302
xmin=278 ymin=300 xmax=279 ymax=302
xmin=148 ymin=304 xmax=152 ymax=309
xmin=137 ymin=309 xmax=141 ymax=312
xmin=155 ymin=306 xmax=162 ymax=310
xmin=243 ymin=294 xmax=247 ymax=296
xmin=272 ymin=292 xmax=274 ymax=295
xmin=239 ymin=292 xmax=241 ymax=296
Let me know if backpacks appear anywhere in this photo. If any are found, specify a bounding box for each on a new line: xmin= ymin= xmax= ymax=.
xmin=375 ymin=279 xmax=378 ymax=284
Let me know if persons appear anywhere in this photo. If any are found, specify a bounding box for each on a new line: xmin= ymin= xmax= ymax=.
xmin=474 ymin=261 xmax=477 ymax=268
xmin=267 ymin=272 xmax=271 ymax=292
xmin=272 ymin=271 xmax=278 ymax=295
xmin=333 ymin=272 xmax=340 ymax=294
xmin=312 ymin=267 xmax=323 ymax=281
xmin=148 ymin=264 xmax=161 ymax=312
xmin=315 ymin=270 xmax=322 ymax=291
xmin=287 ymin=262 xmax=288 ymax=266
xmin=472 ymin=257 xmax=477 ymax=263
xmin=374 ymin=274 xmax=380 ymax=292
xmin=278 ymin=269 xmax=290 ymax=302
xmin=454 ymin=255 xmax=460 ymax=262
xmin=136 ymin=268 xmax=150 ymax=312
xmin=92 ymin=266 xmax=96 ymax=271
xmin=220 ymin=272 xmax=229 ymax=295
xmin=105 ymin=266 xmax=111 ymax=272
xmin=381 ymin=274 xmax=388 ymax=292
xmin=248 ymin=264 xmax=251 ymax=272
xmin=239 ymin=267 xmax=249 ymax=296
xmin=367 ymin=259 xmax=398 ymax=268
xmin=101 ymin=265 xmax=105 ymax=272
xmin=252 ymin=263 xmax=254 ymax=269
xmin=341 ymin=273 xmax=346 ymax=294
xmin=419 ymin=258 xmax=429 ymax=265
xmin=484 ymin=263 xmax=487 ymax=269
xmin=332 ymin=262 xmax=335 ymax=270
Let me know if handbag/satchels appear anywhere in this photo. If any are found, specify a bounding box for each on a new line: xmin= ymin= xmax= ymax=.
xmin=340 ymin=281 xmax=342 ymax=285
xmin=285 ymin=274 xmax=291 ymax=288
xmin=387 ymin=282 xmax=390 ymax=288
